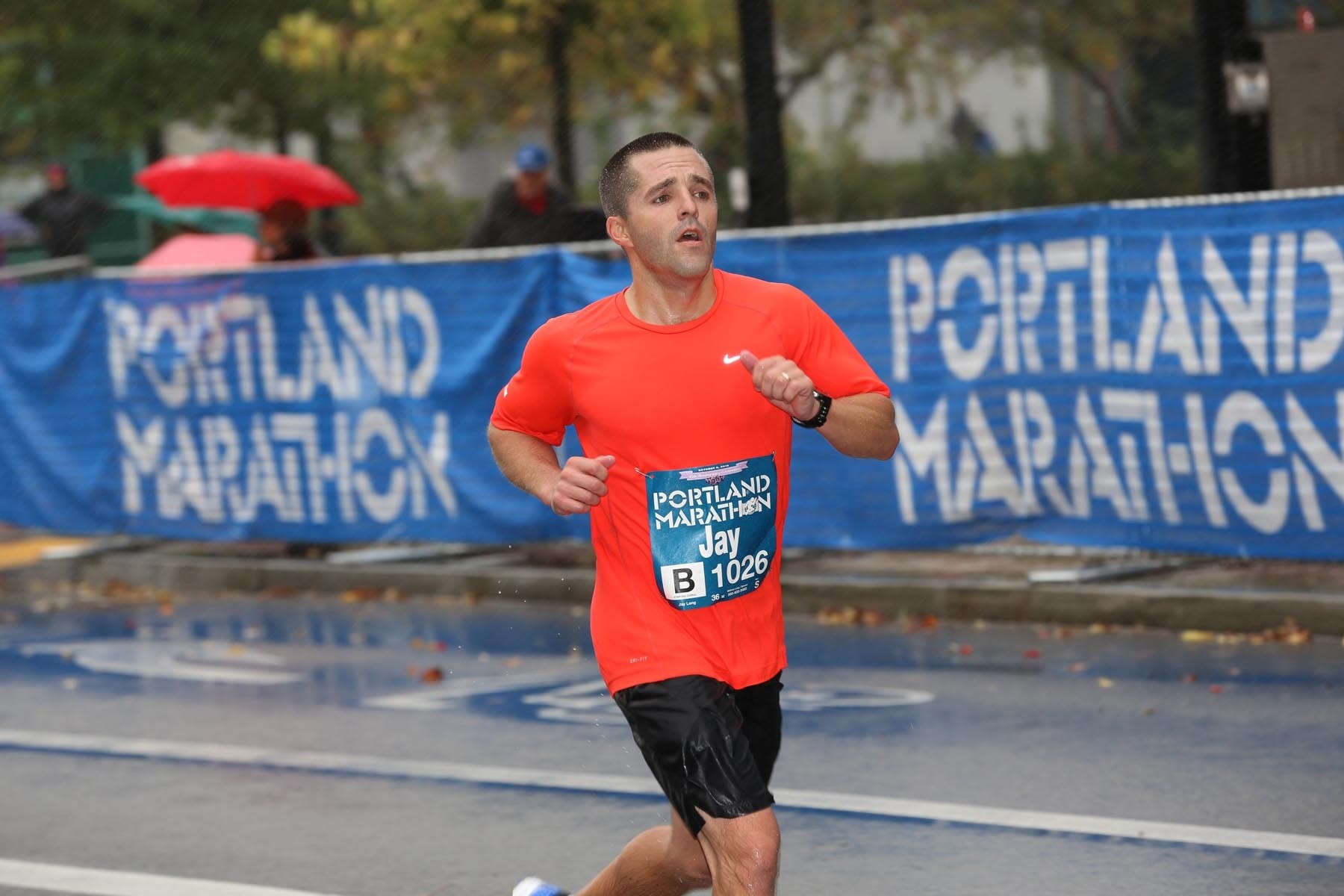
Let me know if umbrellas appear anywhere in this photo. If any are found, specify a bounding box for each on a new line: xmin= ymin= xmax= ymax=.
xmin=140 ymin=232 xmax=275 ymax=272
xmin=136 ymin=152 xmax=361 ymax=263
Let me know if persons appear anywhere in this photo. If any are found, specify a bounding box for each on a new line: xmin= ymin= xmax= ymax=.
xmin=466 ymin=148 xmax=580 ymax=246
xmin=255 ymin=199 xmax=330 ymax=263
xmin=17 ymin=165 xmax=111 ymax=263
xmin=485 ymin=132 xmax=900 ymax=896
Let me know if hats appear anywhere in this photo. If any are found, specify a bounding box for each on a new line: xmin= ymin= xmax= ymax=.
xmin=516 ymin=144 xmax=549 ymax=173
xmin=250 ymin=195 xmax=309 ymax=229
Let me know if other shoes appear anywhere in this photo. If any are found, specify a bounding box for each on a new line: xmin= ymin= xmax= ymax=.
xmin=512 ymin=876 xmax=569 ymax=896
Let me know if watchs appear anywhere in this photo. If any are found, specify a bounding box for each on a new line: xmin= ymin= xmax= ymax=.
xmin=788 ymin=384 xmax=832 ymax=429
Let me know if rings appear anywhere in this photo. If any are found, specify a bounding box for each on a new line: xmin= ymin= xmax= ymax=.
xmin=781 ymin=372 xmax=792 ymax=383
